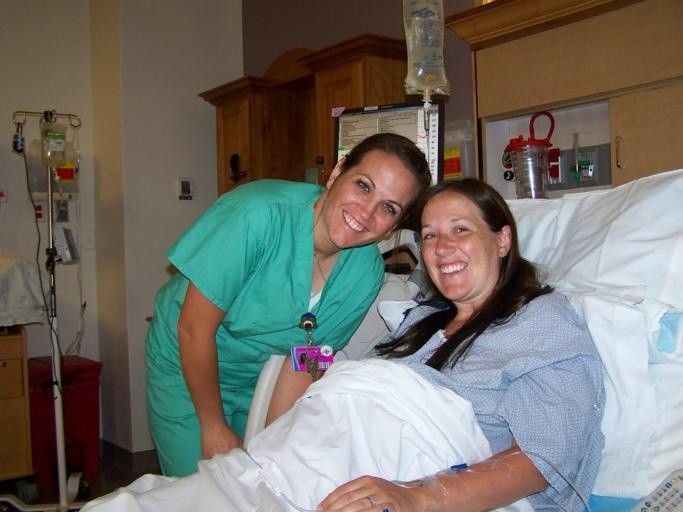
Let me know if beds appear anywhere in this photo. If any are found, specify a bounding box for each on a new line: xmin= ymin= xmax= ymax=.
xmin=0 ymin=169 xmax=683 ymax=512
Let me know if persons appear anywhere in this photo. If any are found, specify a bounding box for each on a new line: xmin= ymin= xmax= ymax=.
xmin=314 ymin=178 xmax=605 ymax=511
xmin=145 ymin=133 xmax=432 ymax=477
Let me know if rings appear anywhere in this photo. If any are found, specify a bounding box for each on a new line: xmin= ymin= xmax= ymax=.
xmin=368 ymin=496 xmax=377 ymax=507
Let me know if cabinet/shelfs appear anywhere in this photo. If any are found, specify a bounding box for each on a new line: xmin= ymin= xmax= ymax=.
xmin=199 ymin=36 xmax=408 ymax=197
xmin=0 ymin=326 xmax=33 ymax=481
xmin=444 ymin=0 xmax=682 ymax=201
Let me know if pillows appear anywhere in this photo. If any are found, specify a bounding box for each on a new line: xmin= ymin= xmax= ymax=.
xmin=378 ymin=284 xmax=646 ymax=500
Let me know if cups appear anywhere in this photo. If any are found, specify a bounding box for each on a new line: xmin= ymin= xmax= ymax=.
xmin=510 ymin=136 xmax=551 ymax=198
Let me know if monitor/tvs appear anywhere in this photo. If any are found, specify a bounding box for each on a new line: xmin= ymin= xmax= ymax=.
xmin=334 ymin=99 xmax=445 ymax=191
